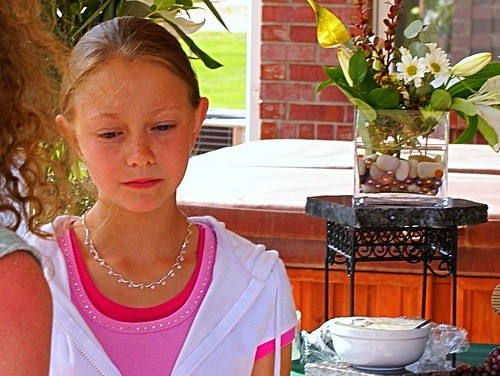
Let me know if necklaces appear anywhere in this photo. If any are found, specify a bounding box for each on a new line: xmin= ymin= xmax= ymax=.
xmin=78 ymin=206 xmax=192 ymax=290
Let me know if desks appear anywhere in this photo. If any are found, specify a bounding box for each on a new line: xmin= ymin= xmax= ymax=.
xmin=290 ymin=331 xmax=500 ymax=376
xmin=304 ymin=195 xmax=488 ymax=368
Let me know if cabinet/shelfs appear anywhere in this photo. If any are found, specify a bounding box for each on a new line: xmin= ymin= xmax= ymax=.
xmin=176 ymin=139 xmax=500 ymax=344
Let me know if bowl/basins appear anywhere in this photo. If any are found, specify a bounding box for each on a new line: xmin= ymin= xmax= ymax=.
xmin=330 ymin=316 xmax=431 ymax=370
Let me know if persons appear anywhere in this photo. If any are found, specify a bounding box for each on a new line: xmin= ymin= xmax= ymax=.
xmin=0 ymin=0 xmax=88 ymax=376
xmin=23 ymin=16 xmax=297 ymax=376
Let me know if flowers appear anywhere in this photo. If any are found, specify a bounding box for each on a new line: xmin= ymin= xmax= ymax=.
xmin=307 ymin=0 xmax=500 ymax=153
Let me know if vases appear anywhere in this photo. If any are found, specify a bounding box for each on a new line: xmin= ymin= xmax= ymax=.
xmin=353 ymin=111 xmax=449 ymax=205
